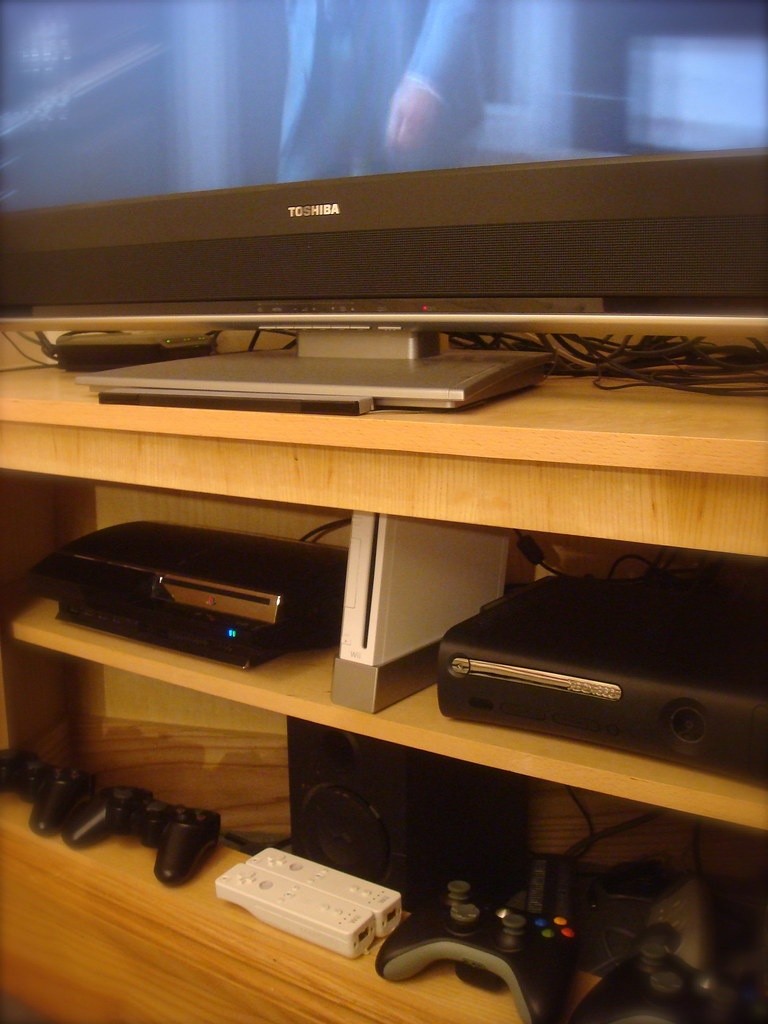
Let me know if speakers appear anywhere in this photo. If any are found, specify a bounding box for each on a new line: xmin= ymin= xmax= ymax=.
xmin=285 ymin=716 xmax=533 ymax=914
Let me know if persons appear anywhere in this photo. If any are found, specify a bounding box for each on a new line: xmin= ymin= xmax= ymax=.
xmin=274 ymin=0 xmax=490 ymax=185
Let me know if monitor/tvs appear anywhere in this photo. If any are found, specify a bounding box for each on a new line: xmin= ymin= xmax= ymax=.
xmin=0 ymin=0 xmax=768 ymax=415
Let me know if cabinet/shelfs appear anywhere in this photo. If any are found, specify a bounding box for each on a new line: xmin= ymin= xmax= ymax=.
xmin=0 ymin=359 xmax=768 ymax=1024
